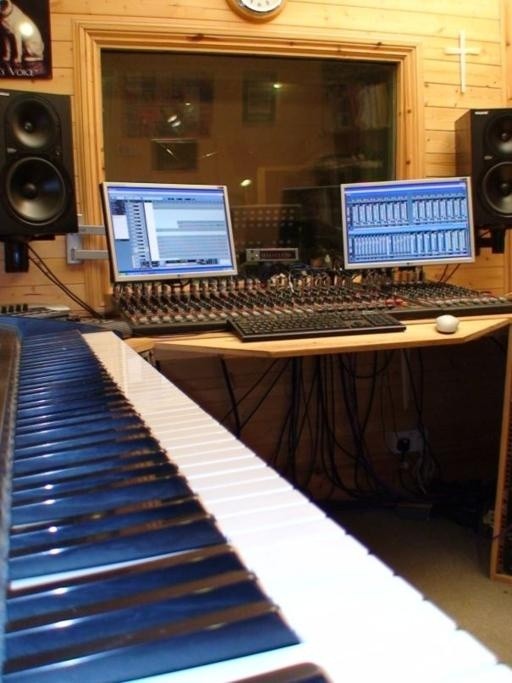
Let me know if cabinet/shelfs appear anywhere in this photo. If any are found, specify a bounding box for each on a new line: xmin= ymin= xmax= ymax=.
xmin=282 ymin=62 xmax=398 ymax=191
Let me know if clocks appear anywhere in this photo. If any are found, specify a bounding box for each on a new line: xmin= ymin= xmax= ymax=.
xmin=224 ymin=0 xmax=289 ymax=23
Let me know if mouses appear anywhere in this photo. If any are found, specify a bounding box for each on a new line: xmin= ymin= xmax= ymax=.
xmin=436 ymin=314 xmax=460 ymax=334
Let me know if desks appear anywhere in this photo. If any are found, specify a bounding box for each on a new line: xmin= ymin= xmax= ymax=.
xmin=120 ymin=309 xmax=512 ymax=358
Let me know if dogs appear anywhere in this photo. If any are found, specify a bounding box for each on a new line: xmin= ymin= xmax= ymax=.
xmin=0 ymin=0 xmax=45 ymax=63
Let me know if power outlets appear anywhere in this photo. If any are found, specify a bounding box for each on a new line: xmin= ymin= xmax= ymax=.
xmin=389 ymin=428 xmax=424 ymax=455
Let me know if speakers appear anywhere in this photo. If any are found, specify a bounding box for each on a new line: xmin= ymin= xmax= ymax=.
xmin=0 ymin=89 xmax=77 ymax=236
xmin=455 ymin=109 xmax=512 ymax=228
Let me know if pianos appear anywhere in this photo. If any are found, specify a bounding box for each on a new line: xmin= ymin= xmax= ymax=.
xmin=0 ymin=313 xmax=512 ymax=683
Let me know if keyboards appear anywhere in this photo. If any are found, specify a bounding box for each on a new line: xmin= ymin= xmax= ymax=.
xmin=228 ymin=310 xmax=406 ymax=342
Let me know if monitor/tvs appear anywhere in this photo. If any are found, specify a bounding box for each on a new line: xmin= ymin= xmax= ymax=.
xmin=98 ymin=182 xmax=239 ymax=283
xmin=339 ymin=176 xmax=475 ymax=269
